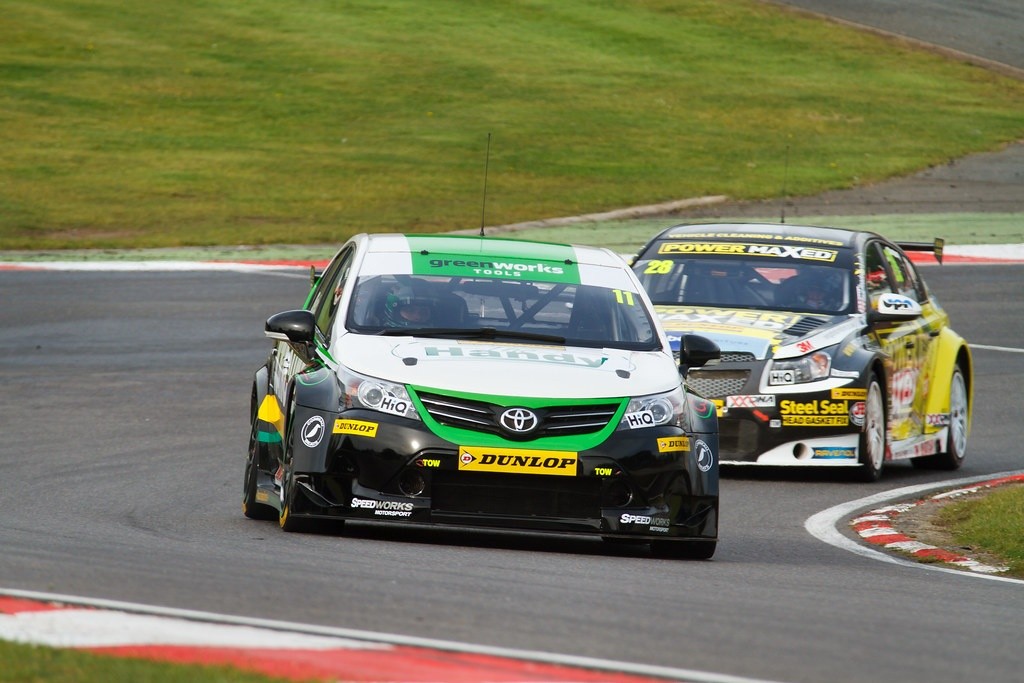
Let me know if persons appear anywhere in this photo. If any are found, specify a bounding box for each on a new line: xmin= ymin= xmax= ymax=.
xmin=385 ymin=277 xmax=442 ymax=329
xmin=791 ymin=271 xmax=840 ymax=311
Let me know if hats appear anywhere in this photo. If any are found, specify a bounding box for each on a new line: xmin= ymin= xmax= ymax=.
xmin=383 ymin=279 xmax=435 ymax=331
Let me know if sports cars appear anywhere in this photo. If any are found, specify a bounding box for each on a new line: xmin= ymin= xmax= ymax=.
xmin=625 ymin=220 xmax=973 ymax=483
xmin=242 ymin=234 xmax=727 ymax=564
